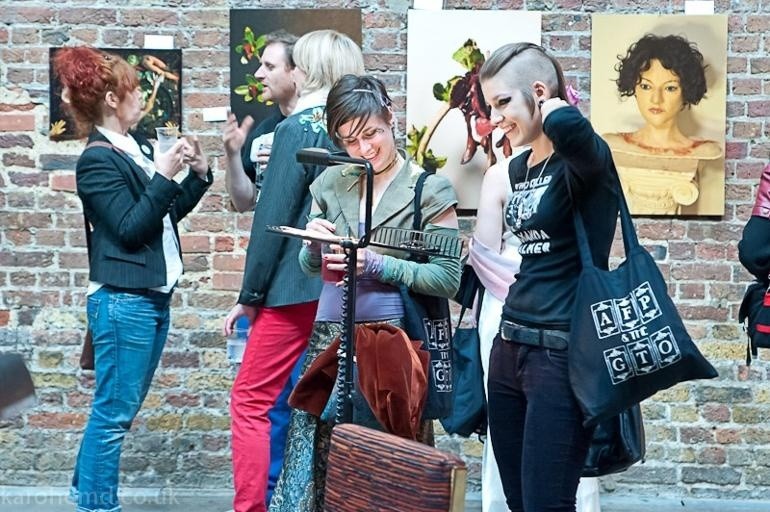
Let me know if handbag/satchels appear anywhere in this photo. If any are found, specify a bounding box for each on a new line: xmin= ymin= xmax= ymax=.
xmin=581 ymin=402 xmax=645 ymax=477
xmin=79 ymin=333 xmax=94 ymax=369
xmin=438 ymin=327 xmax=487 ymax=438
xmin=400 ymin=284 xmax=454 ymax=419
xmin=568 ymin=245 xmax=718 ymax=428
xmin=739 ymin=283 xmax=770 ymax=349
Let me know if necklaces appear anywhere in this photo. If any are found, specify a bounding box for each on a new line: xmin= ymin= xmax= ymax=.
xmin=516 ymin=151 xmax=555 ymax=229
xmin=51 ymin=43 xmax=214 ymax=511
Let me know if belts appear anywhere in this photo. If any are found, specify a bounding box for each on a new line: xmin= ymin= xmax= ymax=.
xmin=499 ymin=319 xmax=569 ymax=350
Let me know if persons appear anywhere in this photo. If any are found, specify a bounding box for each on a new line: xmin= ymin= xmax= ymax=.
xmin=478 ymin=41 xmax=623 ymax=512
xmin=737 ymin=164 xmax=769 ymax=280
xmin=218 ymin=27 xmax=310 ymax=512
xmin=592 ymin=31 xmax=725 ymax=218
xmin=465 ymin=145 xmax=600 ymax=511
xmin=228 ymin=27 xmax=366 ymax=512
xmin=267 ymin=73 xmax=465 ymax=512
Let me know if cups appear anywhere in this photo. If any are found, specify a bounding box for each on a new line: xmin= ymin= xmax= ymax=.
xmin=155 ymin=127 xmax=179 ymax=153
xmin=226 ymin=327 xmax=248 ymax=367
xmin=320 ymin=241 xmax=347 ymax=284
